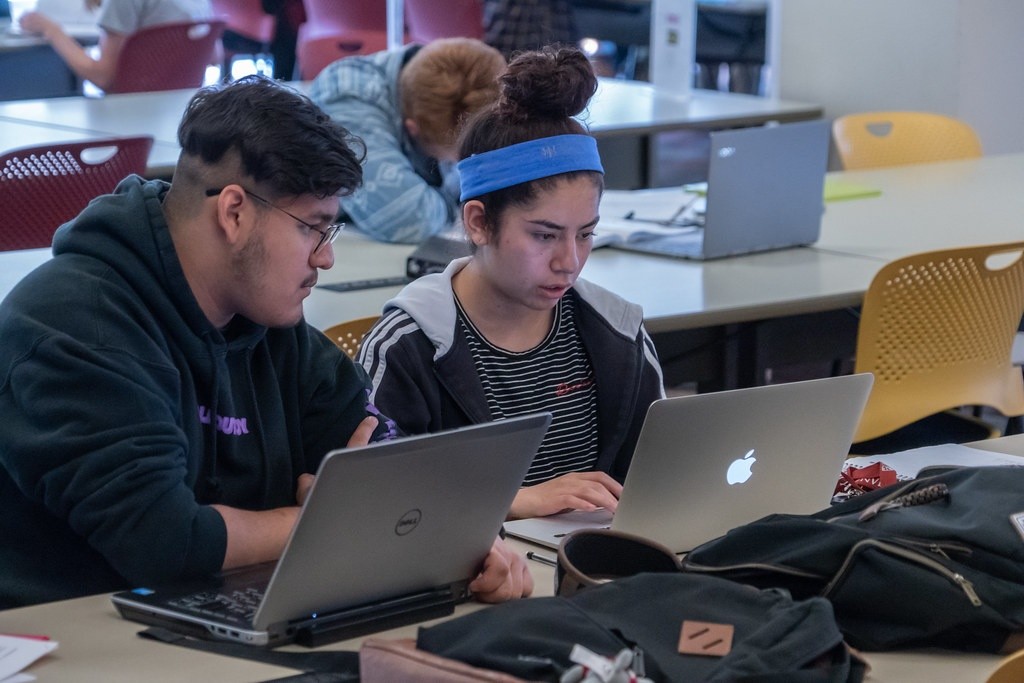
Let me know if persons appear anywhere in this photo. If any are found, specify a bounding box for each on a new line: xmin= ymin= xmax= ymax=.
xmin=0 ymin=79 xmax=534 ymax=606
xmin=300 ymin=40 xmax=507 ymax=245
xmin=354 ymin=44 xmax=669 ymax=518
xmin=21 ymin=0 xmax=224 ymax=91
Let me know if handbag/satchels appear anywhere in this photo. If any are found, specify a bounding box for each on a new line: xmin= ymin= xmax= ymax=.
xmin=361 ymin=571 xmax=872 ymax=683
xmin=682 ymin=465 xmax=1024 ymax=655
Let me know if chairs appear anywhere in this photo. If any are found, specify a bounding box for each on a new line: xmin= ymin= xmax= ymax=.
xmin=836 ymin=111 xmax=980 ymax=172
xmin=0 ymin=0 xmax=484 ymax=240
xmin=854 ymin=241 xmax=1024 ymax=450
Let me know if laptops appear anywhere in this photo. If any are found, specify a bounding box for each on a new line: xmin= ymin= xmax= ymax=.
xmin=609 ymin=117 xmax=831 ymax=260
xmin=502 ymin=373 xmax=875 ymax=556
xmin=109 ymin=412 xmax=553 ymax=648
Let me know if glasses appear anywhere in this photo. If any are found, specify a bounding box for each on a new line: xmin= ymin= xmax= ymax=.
xmin=205 ymin=186 xmax=345 ymax=255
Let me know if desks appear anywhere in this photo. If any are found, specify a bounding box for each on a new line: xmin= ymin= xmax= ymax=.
xmin=2 ymin=434 xmax=1024 ymax=682
xmin=0 ymin=72 xmax=825 ymax=186
xmin=0 ymin=153 xmax=1024 ymax=390
xmin=0 ymin=28 xmax=99 ymax=99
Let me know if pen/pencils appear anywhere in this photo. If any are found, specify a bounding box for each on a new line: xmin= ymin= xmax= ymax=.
xmin=525 ymin=550 xmax=559 ymax=570
